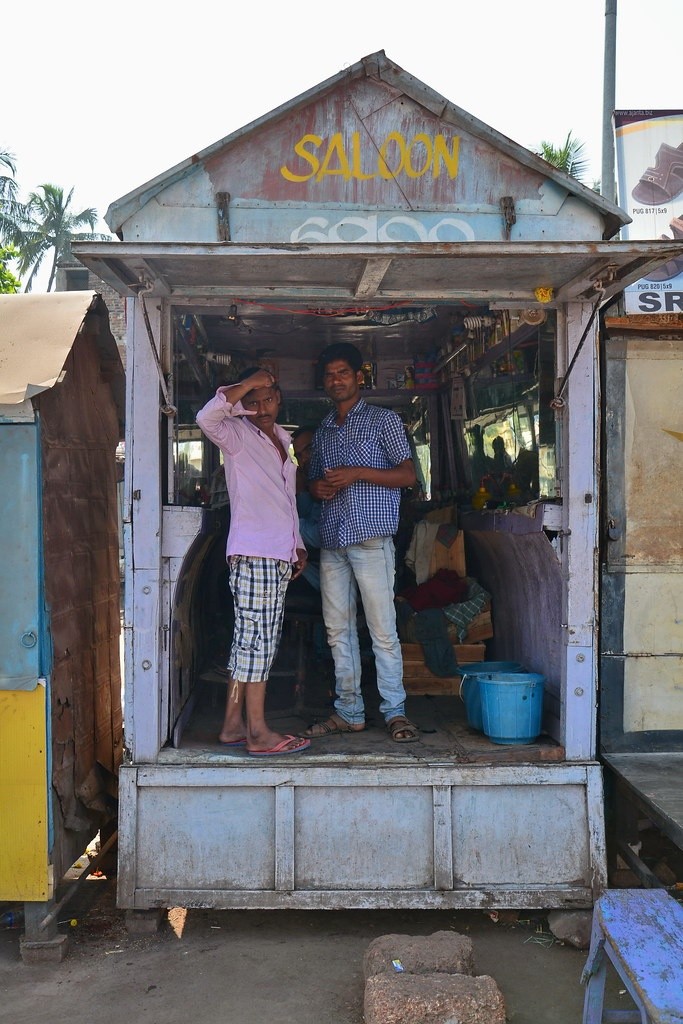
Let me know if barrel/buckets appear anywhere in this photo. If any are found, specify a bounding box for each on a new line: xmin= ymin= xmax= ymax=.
xmin=475 ymin=672 xmax=545 ymax=744
xmin=457 ymin=660 xmax=527 ymax=730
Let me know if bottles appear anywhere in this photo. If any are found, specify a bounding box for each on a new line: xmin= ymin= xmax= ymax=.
xmin=506 ymin=483 xmax=522 ymax=501
xmin=471 ymin=487 xmax=491 ymax=510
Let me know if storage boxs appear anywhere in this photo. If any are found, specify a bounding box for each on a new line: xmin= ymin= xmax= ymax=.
xmin=415 ymin=362 xmax=439 ymax=390
xmin=429 ymin=530 xmax=468 ymax=579
xmin=400 ymin=642 xmax=486 ymax=696
xmin=444 ymin=594 xmax=494 ymax=644
xmin=260 ymin=356 xmax=278 ymax=379
xmin=278 ymin=358 xmax=316 ymax=390
xmin=376 ymin=359 xmax=412 ymax=389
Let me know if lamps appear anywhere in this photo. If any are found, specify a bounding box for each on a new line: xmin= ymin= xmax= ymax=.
xmin=229 ymin=304 xmax=238 ymax=320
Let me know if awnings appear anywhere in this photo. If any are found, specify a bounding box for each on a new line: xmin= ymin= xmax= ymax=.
xmin=70 ymin=238 xmax=683 ymax=415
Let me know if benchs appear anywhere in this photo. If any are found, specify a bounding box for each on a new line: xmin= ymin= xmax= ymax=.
xmin=580 ymin=889 xmax=683 ymax=1024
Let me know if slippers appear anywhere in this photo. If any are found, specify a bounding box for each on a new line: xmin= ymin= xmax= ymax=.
xmin=223 ymin=733 xmax=246 ymax=744
xmin=248 ymin=734 xmax=309 ymax=756
xmin=388 ymin=715 xmax=418 ymax=741
xmin=298 ymin=714 xmax=350 ymax=739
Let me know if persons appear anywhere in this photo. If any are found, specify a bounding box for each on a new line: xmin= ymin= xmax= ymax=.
xmin=306 ymin=343 xmax=420 ymax=742
xmin=196 ymin=367 xmax=310 ymax=755
xmin=287 ymin=426 xmax=321 ymax=611
